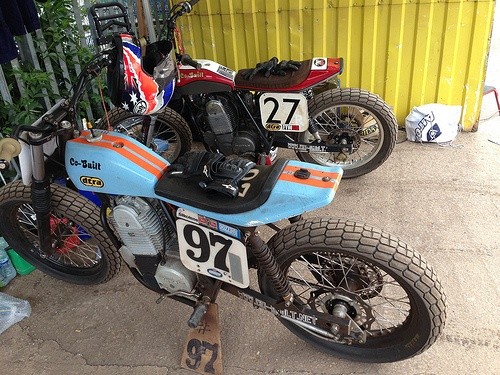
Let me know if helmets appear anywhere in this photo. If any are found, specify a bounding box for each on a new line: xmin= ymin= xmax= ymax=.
xmin=106 ymin=32 xmax=179 ymax=115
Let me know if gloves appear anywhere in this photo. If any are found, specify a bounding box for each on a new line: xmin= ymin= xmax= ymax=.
xmin=183 ymin=151 xmax=229 ymax=190
xmin=272 ymin=60 xmax=301 ymax=76
xmin=208 ymin=154 xmax=255 ymax=197
xmin=240 ymin=57 xmax=278 ymax=80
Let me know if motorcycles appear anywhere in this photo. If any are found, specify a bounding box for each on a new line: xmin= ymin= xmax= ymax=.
xmin=76 ymin=0 xmax=397 ymax=182
xmin=0 ymin=51 xmax=451 ymax=375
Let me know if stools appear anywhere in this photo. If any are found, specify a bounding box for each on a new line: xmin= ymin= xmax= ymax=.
xmin=482 ymin=84 xmax=500 ymax=117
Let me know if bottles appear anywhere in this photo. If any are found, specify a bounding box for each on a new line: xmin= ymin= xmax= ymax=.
xmin=0 ymin=236 xmax=17 ymax=289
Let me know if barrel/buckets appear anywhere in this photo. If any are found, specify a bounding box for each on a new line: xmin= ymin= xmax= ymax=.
xmin=6 ymin=247 xmax=37 ymax=276
